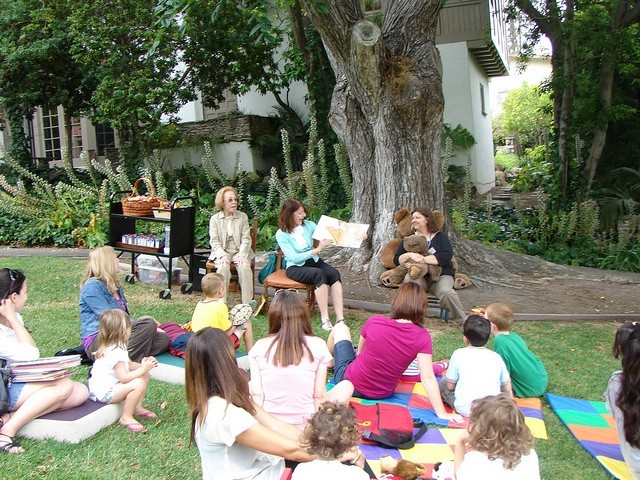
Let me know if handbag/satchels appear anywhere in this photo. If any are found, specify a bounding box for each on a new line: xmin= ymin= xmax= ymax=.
xmin=258 ymin=243 xmax=287 ymax=284
xmin=348 ymin=401 xmax=427 ymax=449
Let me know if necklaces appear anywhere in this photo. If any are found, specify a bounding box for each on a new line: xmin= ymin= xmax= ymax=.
xmin=418 ymin=232 xmax=432 ymax=249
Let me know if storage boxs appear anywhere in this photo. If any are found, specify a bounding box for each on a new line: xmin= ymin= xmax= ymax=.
xmin=193 ymin=250 xmax=239 ymax=292
xmin=138 ymin=263 xmax=184 ymax=287
xmin=134 ymin=253 xmax=179 ymax=283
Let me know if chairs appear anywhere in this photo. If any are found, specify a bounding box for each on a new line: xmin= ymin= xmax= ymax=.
xmin=263 ymin=239 xmax=320 ymax=317
xmin=204 ymin=215 xmax=259 ymax=304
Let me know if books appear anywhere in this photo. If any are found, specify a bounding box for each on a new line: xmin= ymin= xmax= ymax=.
xmin=311 ymin=214 xmax=370 ymax=249
xmin=10 ymin=354 xmax=82 ymax=383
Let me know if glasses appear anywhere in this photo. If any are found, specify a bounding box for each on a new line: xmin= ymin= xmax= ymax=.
xmin=3 ymin=269 xmax=19 ymax=304
xmin=228 ymin=198 xmax=238 ymax=202
xmin=275 ymin=287 xmax=299 ymax=294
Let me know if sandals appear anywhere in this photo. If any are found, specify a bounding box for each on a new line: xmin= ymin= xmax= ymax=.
xmin=0 ymin=433 xmax=26 ymax=454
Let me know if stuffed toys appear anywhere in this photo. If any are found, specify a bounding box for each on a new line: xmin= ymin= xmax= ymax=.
xmin=380 ymin=208 xmax=470 ymax=289
xmin=380 ymin=235 xmax=442 ymax=287
xmin=432 ymin=460 xmax=456 ymax=480
xmin=380 ymin=454 xmax=427 ymax=480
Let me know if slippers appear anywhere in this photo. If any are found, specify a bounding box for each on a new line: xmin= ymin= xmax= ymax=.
xmin=137 ymin=410 xmax=157 ymax=419
xmin=119 ymin=421 xmax=145 ymax=432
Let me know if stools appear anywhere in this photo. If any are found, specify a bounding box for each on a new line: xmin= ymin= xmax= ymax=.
xmin=424 ymin=292 xmax=450 ymax=324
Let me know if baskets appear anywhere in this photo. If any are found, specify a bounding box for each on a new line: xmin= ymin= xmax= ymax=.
xmin=121 ymin=177 xmax=166 ymax=217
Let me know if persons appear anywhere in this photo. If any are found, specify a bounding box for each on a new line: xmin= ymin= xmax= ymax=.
xmin=138 ymin=315 xmax=189 ymax=359
xmin=290 ymin=400 xmax=370 ymax=480
xmin=275 ymin=199 xmax=368 ymax=330
xmin=326 ymin=281 xmax=464 ymax=423
xmin=454 ymin=395 xmax=540 ymax=480
xmin=88 ymin=309 xmax=158 ymax=431
xmin=604 ymin=321 xmax=640 ymax=479
xmin=80 ymin=245 xmax=169 ymax=364
xmin=208 ymin=186 xmax=255 ymax=305
xmin=393 ymin=207 xmax=467 ymax=333
xmin=484 ymin=303 xmax=548 ymax=397
xmin=185 ymin=326 xmax=380 ymax=480
xmin=248 ymin=289 xmax=354 ymax=430
xmin=192 ymin=273 xmax=253 ymax=352
xmin=0 ymin=268 xmax=89 ymax=455
xmin=440 ymin=315 xmax=513 ymax=417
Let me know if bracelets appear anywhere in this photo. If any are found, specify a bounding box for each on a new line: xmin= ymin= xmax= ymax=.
xmin=349 ymin=450 xmax=362 ymax=464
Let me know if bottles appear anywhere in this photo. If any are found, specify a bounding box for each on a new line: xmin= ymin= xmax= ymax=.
xmin=121 ymin=234 xmax=164 ymax=248
